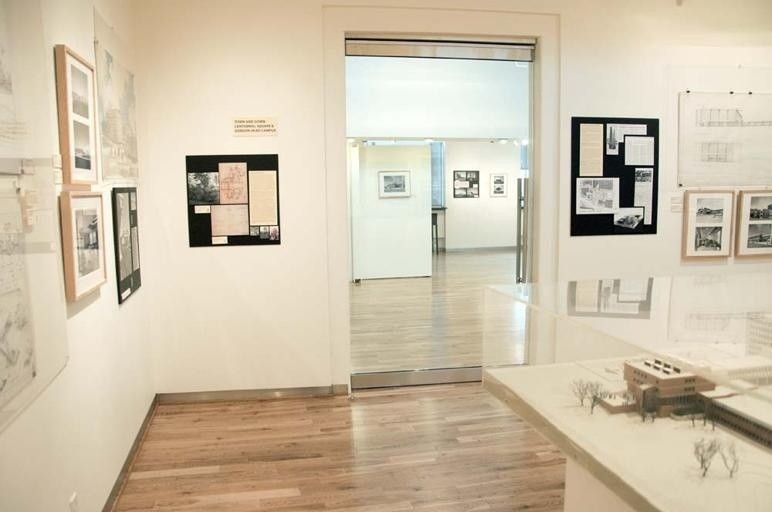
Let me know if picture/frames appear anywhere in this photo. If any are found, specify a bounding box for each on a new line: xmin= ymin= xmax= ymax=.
xmin=56 ymin=189 xmax=109 ymax=305
xmin=677 ymin=187 xmax=735 ymax=261
xmin=490 ymin=173 xmax=508 ymax=198
xmin=732 ymin=188 xmax=772 ymax=259
xmin=53 ymin=44 xmax=102 ymax=188
xmin=377 ymin=168 xmax=411 ymax=200
